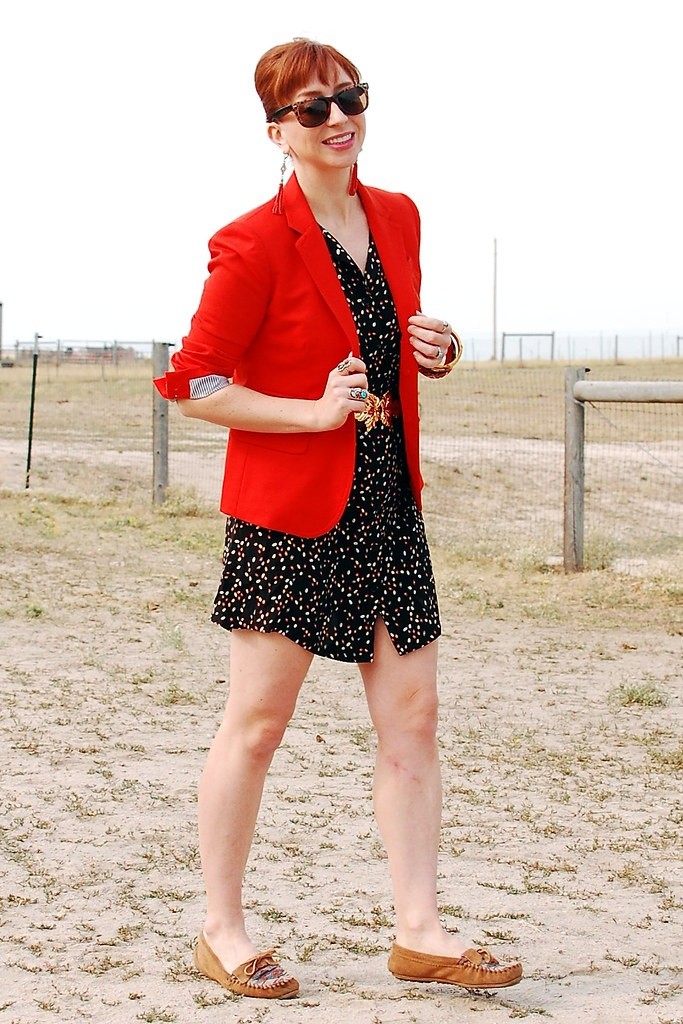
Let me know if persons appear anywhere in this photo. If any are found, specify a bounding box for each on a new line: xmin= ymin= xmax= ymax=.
xmin=151 ymin=36 xmax=521 ymax=1000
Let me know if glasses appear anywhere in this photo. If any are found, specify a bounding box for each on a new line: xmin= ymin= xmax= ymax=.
xmin=270 ymin=82 xmax=369 ymax=128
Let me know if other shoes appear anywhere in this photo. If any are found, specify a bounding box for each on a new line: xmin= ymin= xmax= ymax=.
xmin=193 ymin=929 xmax=299 ymax=999
xmin=388 ymin=943 xmax=523 ymax=988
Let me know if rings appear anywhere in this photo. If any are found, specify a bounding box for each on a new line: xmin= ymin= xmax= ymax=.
xmin=337 ymin=358 xmax=351 ymax=373
xmin=436 ymin=347 xmax=442 ymax=358
xmin=349 ymin=387 xmax=370 ymax=400
xmin=442 ymin=321 xmax=448 ymax=332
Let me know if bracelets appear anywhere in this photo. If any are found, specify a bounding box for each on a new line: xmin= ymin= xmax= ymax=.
xmin=430 ymin=330 xmax=463 ymax=372
